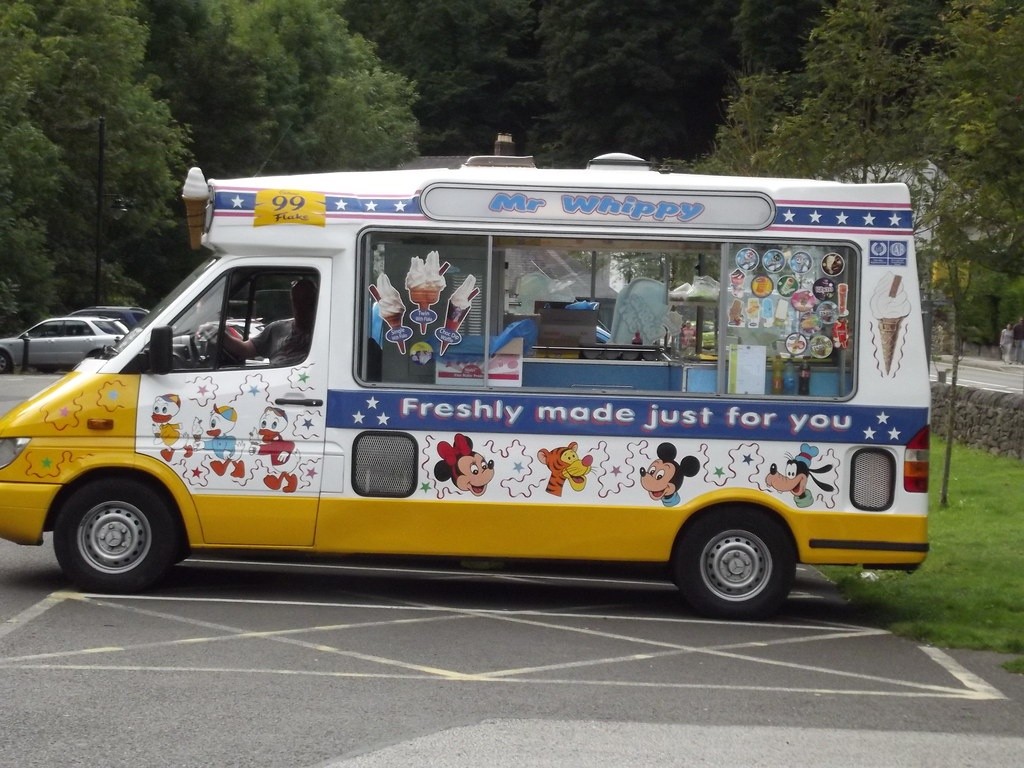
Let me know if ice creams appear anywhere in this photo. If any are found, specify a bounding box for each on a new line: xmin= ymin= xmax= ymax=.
xmin=369 ymin=251 xmax=479 ymax=356
xmin=182 ymin=166 xmax=211 ymax=250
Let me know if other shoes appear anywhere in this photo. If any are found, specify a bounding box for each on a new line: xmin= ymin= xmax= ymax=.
xmin=1015 ymin=361 xmax=1023 ymax=365
xmin=1004 ymin=361 xmax=1012 ymax=364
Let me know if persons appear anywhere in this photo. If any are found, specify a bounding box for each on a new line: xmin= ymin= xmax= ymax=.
xmin=999 ymin=318 xmax=1024 ymax=365
xmin=199 ymin=278 xmax=318 ymax=367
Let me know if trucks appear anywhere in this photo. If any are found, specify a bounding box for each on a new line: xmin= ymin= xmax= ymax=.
xmin=0 ymin=132 xmax=930 ymax=623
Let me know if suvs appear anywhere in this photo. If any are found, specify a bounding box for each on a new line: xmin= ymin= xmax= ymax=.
xmin=0 ymin=317 xmax=129 ymax=374
xmin=69 ymin=306 xmax=149 ymax=332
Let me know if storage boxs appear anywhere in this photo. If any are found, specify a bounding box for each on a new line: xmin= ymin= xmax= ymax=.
xmin=536 ymin=306 xmax=599 ymax=343
xmin=434 ymin=335 xmax=523 ymax=387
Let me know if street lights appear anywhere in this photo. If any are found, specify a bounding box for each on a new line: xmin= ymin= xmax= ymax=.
xmin=94 ymin=192 xmax=129 ymax=305
